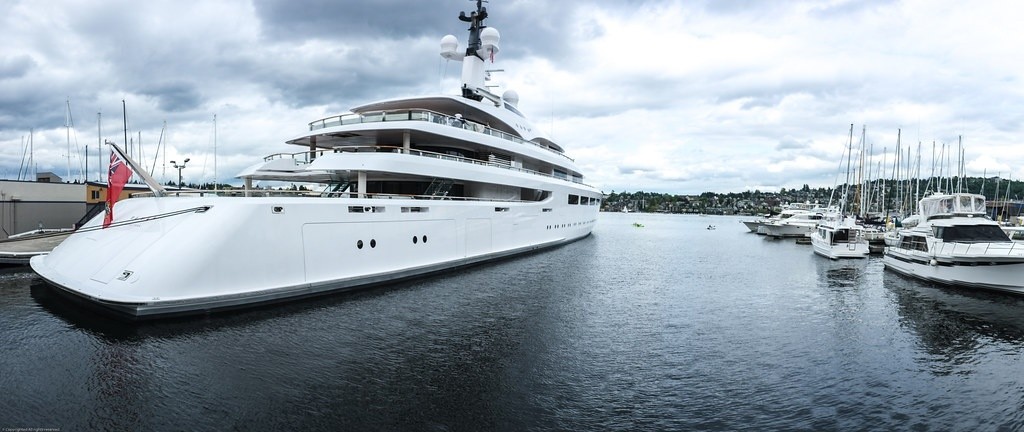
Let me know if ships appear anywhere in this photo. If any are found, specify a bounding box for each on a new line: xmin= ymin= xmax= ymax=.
xmin=28 ymin=0 xmax=602 ymax=318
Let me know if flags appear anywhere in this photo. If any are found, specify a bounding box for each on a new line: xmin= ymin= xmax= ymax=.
xmin=102 ymin=149 xmax=133 ymax=229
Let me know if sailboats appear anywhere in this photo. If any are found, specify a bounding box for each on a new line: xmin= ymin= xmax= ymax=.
xmin=739 ymin=201 xmax=1024 ymax=260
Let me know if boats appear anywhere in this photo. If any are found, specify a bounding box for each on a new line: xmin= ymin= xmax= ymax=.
xmin=706 ymin=224 xmax=716 ymax=230
xmin=632 ymin=222 xmax=645 ymax=228
xmin=0 ymin=219 xmax=75 ymax=265
xmin=881 ymin=193 xmax=1024 ymax=300
